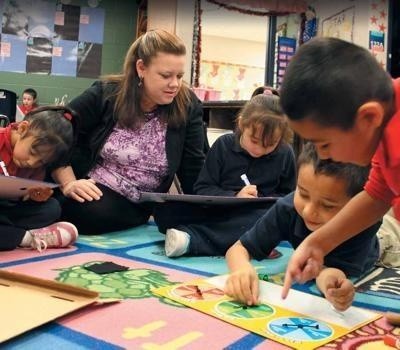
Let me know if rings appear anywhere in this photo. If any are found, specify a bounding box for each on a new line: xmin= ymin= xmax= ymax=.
xmin=36 ymin=192 xmax=42 ymax=196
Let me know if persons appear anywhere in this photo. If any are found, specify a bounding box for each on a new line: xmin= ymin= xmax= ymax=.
xmin=224 ymin=141 xmax=400 ymax=311
xmin=279 ymin=36 xmax=400 ymax=300
xmin=0 ymin=105 xmax=79 ymax=252
xmin=18 ymin=89 xmax=37 ymax=116
xmin=152 ymin=86 xmax=296 ymax=257
xmin=45 ymin=28 xmax=210 ymax=235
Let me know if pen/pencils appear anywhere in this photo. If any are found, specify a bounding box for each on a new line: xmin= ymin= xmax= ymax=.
xmin=0 ymin=161 xmax=9 ymax=177
xmin=240 ymin=173 xmax=251 ymax=185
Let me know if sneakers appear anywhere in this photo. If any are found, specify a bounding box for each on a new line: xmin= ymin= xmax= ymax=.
xmin=24 ymin=221 xmax=78 ymax=253
xmin=166 ymin=228 xmax=190 ymax=257
